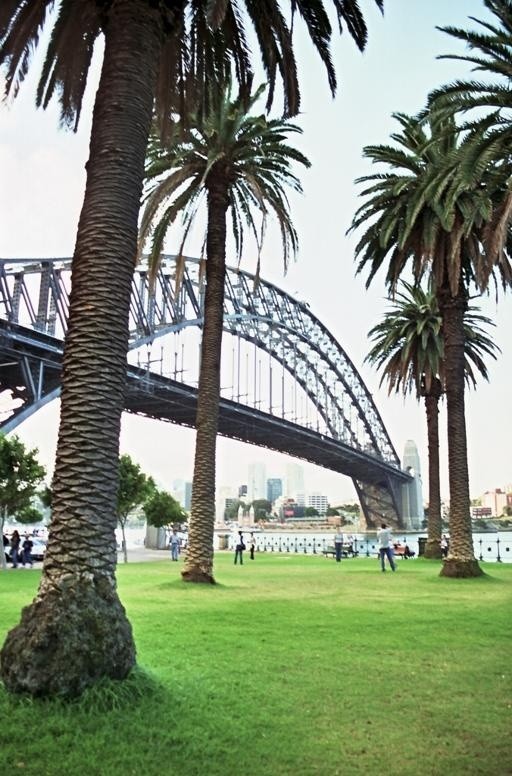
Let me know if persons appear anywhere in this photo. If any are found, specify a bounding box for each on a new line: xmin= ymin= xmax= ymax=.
xmin=169 ymin=530 xmax=180 ymax=562
xmin=10 ymin=530 xmax=21 ymax=568
xmin=233 ymin=531 xmax=244 ymax=565
xmin=377 ymin=524 xmax=395 ymax=572
xmin=22 ymin=536 xmax=34 ymax=567
xmin=248 ymin=532 xmax=256 ymax=560
xmin=333 ymin=526 xmax=343 ymax=562
xmin=441 ymin=535 xmax=448 ymax=553
xmin=391 ymin=539 xmax=415 ymax=560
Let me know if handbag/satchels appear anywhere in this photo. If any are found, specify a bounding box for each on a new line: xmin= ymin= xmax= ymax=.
xmin=237 ymin=543 xmax=245 ymax=551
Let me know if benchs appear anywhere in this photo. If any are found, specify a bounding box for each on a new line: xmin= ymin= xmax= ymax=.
xmin=392 ymin=545 xmax=408 ymax=560
xmin=321 ymin=545 xmax=359 ymax=558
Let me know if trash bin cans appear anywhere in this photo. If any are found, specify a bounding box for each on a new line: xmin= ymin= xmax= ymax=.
xmin=218 ymin=534 xmax=230 ymax=550
xmin=418 ymin=537 xmax=427 ymax=557
